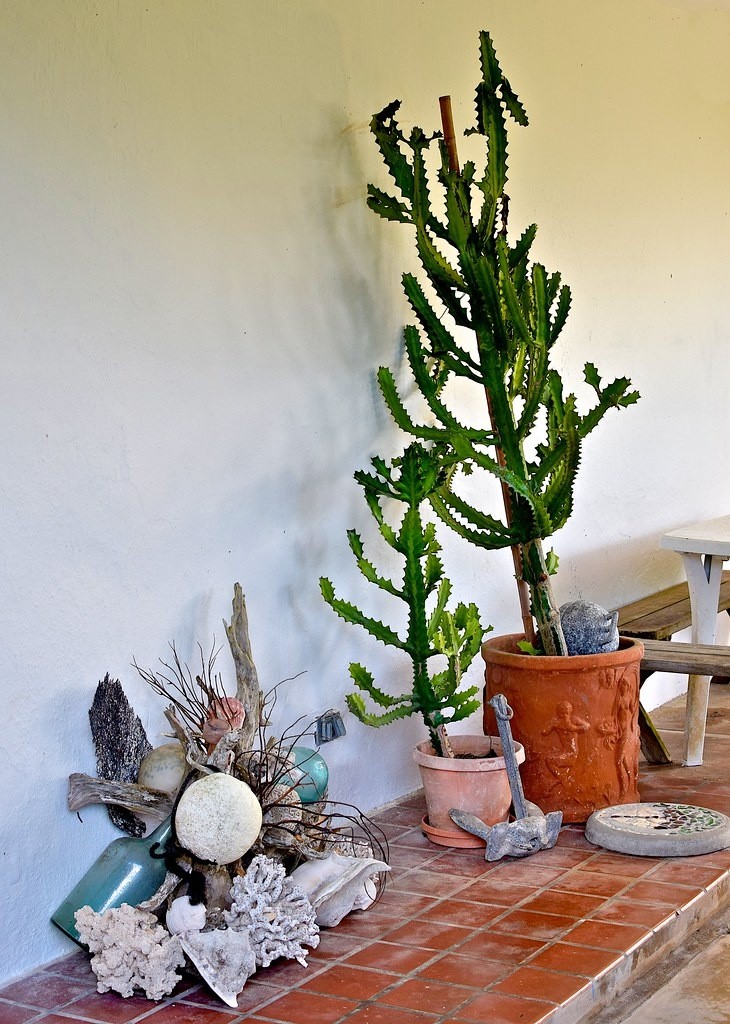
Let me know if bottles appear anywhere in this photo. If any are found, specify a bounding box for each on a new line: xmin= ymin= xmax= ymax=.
xmin=50 ymin=814 xmax=173 ymax=957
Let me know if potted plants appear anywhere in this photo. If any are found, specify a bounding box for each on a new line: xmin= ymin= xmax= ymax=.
xmin=367 ymin=28 xmax=643 ymax=826
xmin=320 ymin=443 xmax=526 ymax=850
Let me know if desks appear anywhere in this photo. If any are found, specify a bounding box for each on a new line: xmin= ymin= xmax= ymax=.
xmin=658 ymin=514 xmax=730 ymax=767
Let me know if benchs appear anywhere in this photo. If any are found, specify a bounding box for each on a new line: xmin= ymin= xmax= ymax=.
xmin=607 ymin=570 xmax=730 ymax=764
